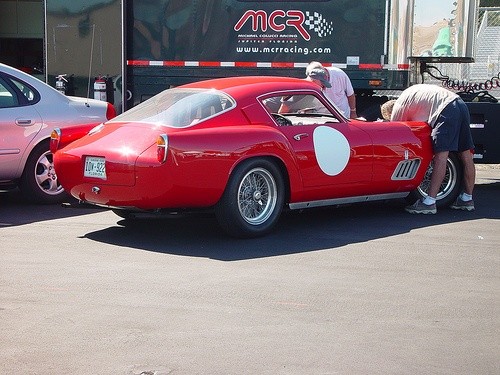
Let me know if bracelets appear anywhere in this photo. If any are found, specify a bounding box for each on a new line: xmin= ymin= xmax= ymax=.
xmin=350 ymin=109 xmax=356 ymax=112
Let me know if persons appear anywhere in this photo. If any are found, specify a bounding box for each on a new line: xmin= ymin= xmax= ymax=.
xmin=306 ymin=61 xmax=357 ymax=119
xmin=381 ymin=84 xmax=476 ymax=214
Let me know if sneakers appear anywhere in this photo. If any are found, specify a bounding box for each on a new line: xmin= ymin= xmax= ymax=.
xmin=405 ymin=197 xmax=437 ymax=214
xmin=449 ymin=197 xmax=474 ymax=211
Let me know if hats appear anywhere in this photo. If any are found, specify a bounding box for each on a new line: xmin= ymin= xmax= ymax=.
xmin=310 ymin=69 xmax=332 ymax=88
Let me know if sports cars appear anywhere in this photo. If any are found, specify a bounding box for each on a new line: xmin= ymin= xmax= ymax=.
xmin=49 ymin=75 xmax=464 ymax=236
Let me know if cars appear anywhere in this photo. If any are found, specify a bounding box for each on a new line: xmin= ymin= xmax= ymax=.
xmin=0 ymin=63 xmax=117 ymax=204
